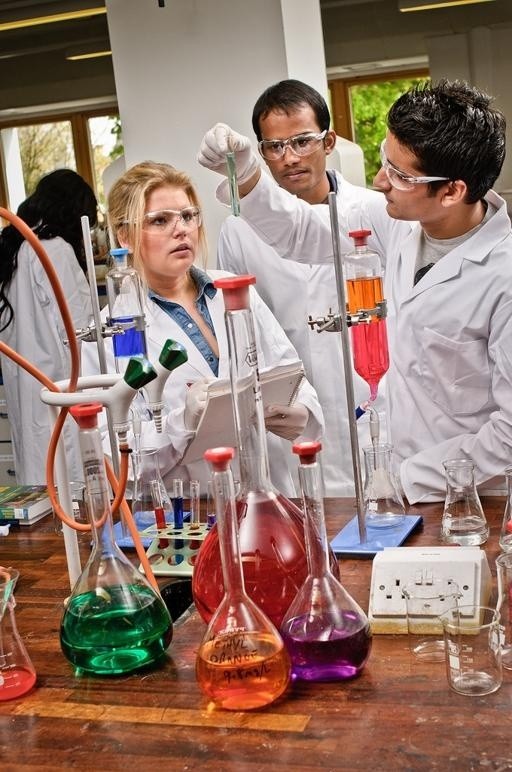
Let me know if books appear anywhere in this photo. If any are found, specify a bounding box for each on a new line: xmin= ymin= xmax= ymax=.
xmin=0 ymin=486 xmax=52 ymax=526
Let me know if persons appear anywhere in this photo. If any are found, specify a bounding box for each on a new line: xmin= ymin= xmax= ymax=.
xmin=0 ymin=168 xmax=96 ymax=500
xmin=198 ymin=78 xmax=512 ymax=504
xmin=216 ymin=80 xmax=385 ymax=498
xmin=81 ymin=161 xmax=324 ymax=499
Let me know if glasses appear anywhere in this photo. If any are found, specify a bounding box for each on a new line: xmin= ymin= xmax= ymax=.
xmin=257 ymin=127 xmax=329 ymax=162
xmin=377 ymin=136 xmax=451 ymax=193
xmin=124 ymin=205 xmax=203 ymax=237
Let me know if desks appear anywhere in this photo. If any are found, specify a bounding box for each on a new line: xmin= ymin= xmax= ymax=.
xmin=0 ymin=492 xmax=510 ymax=772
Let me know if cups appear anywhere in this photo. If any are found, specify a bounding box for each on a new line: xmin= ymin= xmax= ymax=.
xmin=399 ymin=577 xmax=504 ymax=697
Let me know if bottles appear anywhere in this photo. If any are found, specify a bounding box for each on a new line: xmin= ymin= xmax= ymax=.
xmin=361 ymin=446 xmax=511 ymax=555
xmin=343 ymin=231 xmax=390 ymax=401
xmin=0 ymin=569 xmax=38 ymax=701
xmin=184 ymin=277 xmax=340 ymax=654
xmin=280 ymin=441 xmax=373 ymax=684
xmin=125 ymin=442 xmax=248 ymax=552
xmin=224 ymin=152 xmax=242 ymax=217
xmin=189 ymin=451 xmax=294 ymax=713
xmin=56 ymin=405 xmax=175 ymax=678
xmin=102 ymin=242 xmax=153 ymax=441
xmin=488 ymin=549 xmax=512 ymax=673
